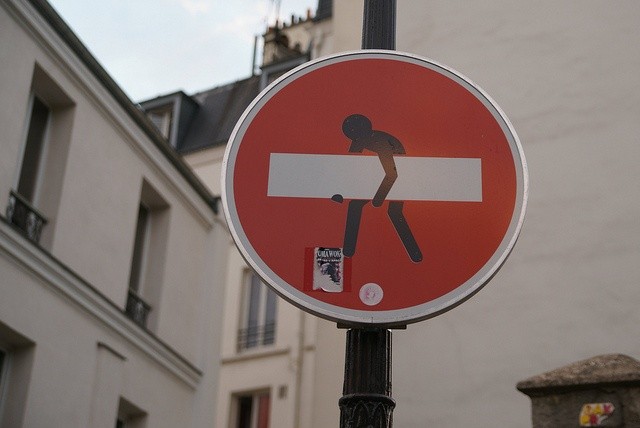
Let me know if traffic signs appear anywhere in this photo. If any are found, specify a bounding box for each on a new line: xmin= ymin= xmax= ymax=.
xmin=221 ymin=48 xmax=529 ymax=329
